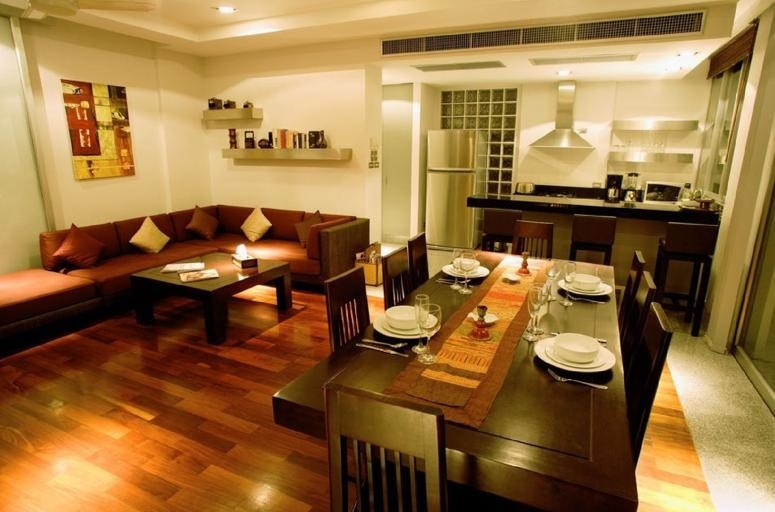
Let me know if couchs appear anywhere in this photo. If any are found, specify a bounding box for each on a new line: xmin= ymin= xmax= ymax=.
xmin=39 ymin=205 xmax=370 ymax=307
xmin=0 ymin=268 xmax=101 ymax=342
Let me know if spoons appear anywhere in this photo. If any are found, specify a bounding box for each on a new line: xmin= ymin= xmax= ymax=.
xmin=361 ymin=338 xmax=408 ymax=349
xmin=438 ymin=278 xmax=472 ymax=284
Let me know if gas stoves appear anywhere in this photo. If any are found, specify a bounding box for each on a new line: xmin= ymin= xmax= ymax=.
xmin=513 ymin=190 xmax=579 ymax=198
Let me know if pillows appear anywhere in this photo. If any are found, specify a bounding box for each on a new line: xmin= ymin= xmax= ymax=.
xmin=129 ymin=213 xmax=170 ymax=254
xmin=294 ymin=210 xmax=322 ymax=248
xmin=52 ymin=223 xmax=107 ymax=269
xmin=185 ymin=206 xmax=219 ymax=240
xmin=239 ymin=207 xmax=272 ymax=242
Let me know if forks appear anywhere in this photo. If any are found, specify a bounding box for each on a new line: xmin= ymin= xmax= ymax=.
xmin=546 ymin=367 xmax=609 ymax=389
xmin=564 ymin=292 xmax=607 ymax=305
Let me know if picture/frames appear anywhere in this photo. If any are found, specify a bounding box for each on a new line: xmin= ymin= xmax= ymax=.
xmin=57 ymin=75 xmax=138 ymax=182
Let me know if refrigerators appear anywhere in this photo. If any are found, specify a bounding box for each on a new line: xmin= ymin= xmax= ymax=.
xmin=423 ymin=128 xmax=478 ymax=252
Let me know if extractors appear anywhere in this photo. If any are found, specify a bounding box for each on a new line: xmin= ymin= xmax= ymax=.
xmin=528 ymin=80 xmax=596 ymax=149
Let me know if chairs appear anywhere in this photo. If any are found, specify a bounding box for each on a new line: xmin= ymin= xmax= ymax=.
xmin=408 ymin=232 xmax=429 ymax=291
xmin=617 ymin=271 xmax=656 ymax=375
xmin=617 ymin=250 xmax=646 ymax=315
xmin=512 ymin=220 xmax=554 ymax=259
xmin=482 ymin=208 xmax=523 ymax=254
xmin=654 ymin=222 xmax=720 ymax=337
xmin=570 ymin=213 xmax=617 ymax=267
xmin=625 ymin=302 xmax=674 ymax=468
xmin=323 ymin=265 xmax=370 ymax=353
xmin=381 ymin=247 xmax=412 ymax=311
xmin=321 ymin=381 xmax=448 ymax=512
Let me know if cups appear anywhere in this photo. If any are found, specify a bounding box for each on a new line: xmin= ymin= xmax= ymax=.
xmin=411 ymin=293 xmax=431 ymax=355
xmin=523 ymin=287 xmax=543 ymax=342
xmin=417 ymin=303 xmax=442 ymax=365
xmin=559 ymin=261 xmax=577 ymax=307
xmin=592 ymin=183 xmax=601 ymax=200
xmin=460 ymin=250 xmax=477 ymax=295
xmin=545 ymin=257 xmax=562 ymax=302
xmin=526 ymin=282 xmax=551 ymax=335
xmin=451 ymin=247 xmax=467 ymax=291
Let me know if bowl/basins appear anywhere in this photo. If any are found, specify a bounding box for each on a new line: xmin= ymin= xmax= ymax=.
xmin=453 ymin=258 xmax=480 ymax=271
xmin=572 ymin=273 xmax=602 ymax=290
xmin=384 ymin=306 xmax=420 ymax=330
xmin=553 ymin=332 xmax=599 ymax=363
xmin=516 ymin=181 xmax=536 ymax=194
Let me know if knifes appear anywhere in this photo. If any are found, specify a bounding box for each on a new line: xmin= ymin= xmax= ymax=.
xmin=354 ymin=343 xmax=410 ymax=358
xmin=550 ymin=332 xmax=607 ymax=344
xmin=435 ymin=279 xmax=474 ymax=288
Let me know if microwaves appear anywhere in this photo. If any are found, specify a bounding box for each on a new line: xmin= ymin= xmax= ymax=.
xmin=643 ymin=181 xmax=685 ymax=205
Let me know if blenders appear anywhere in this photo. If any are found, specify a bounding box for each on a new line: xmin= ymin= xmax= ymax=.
xmin=624 ymin=171 xmax=640 ymax=202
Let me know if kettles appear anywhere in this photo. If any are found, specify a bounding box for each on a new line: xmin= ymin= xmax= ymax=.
xmin=606 ymin=186 xmax=620 ymax=199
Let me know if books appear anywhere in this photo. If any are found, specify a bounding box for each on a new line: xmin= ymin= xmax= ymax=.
xmin=160 ymin=263 xmax=205 ymax=272
xmin=178 ymin=269 xmax=220 ymax=282
xmin=271 ymin=128 xmax=305 ymax=149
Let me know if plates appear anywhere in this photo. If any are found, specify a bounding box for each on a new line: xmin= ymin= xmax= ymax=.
xmin=373 ymin=314 xmax=442 ymax=340
xmin=441 ymin=264 xmax=490 ymax=278
xmin=382 ymin=319 xmax=420 ymax=335
xmin=449 ymin=267 xmax=485 ymax=275
xmin=567 ymin=284 xmax=606 ymax=293
xmin=466 ymin=312 xmax=499 ymax=324
xmin=544 ymin=342 xmax=610 ymax=368
xmin=557 ymin=278 xmax=615 ymax=296
xmin=533 ymin=337 xmax=618 ymax=373
xmin=502 ymin=273 xmax=522 ymax=282
